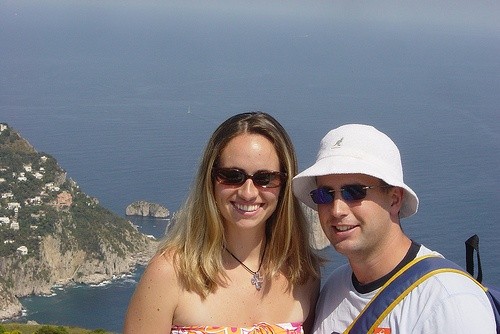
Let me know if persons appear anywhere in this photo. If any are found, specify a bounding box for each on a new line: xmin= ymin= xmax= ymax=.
xmin=290 ymin=123 xmax=500 ymax=334
xmin=123 ymin=112 xmax=322 ymax=334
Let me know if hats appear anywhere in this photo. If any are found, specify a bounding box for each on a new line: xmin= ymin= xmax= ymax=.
xmin=291 ymin=124 xmax=419 ymax=218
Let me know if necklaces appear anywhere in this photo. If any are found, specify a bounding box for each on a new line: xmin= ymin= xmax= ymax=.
xmin=225 ymin=235 xmax=268 ymax=290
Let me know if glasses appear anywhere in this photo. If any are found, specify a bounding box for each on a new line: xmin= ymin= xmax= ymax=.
xmin=211 ymin=167 xmax=287 ymax=188
xmin=307 ymin=184 xmax=391 ymax=204
